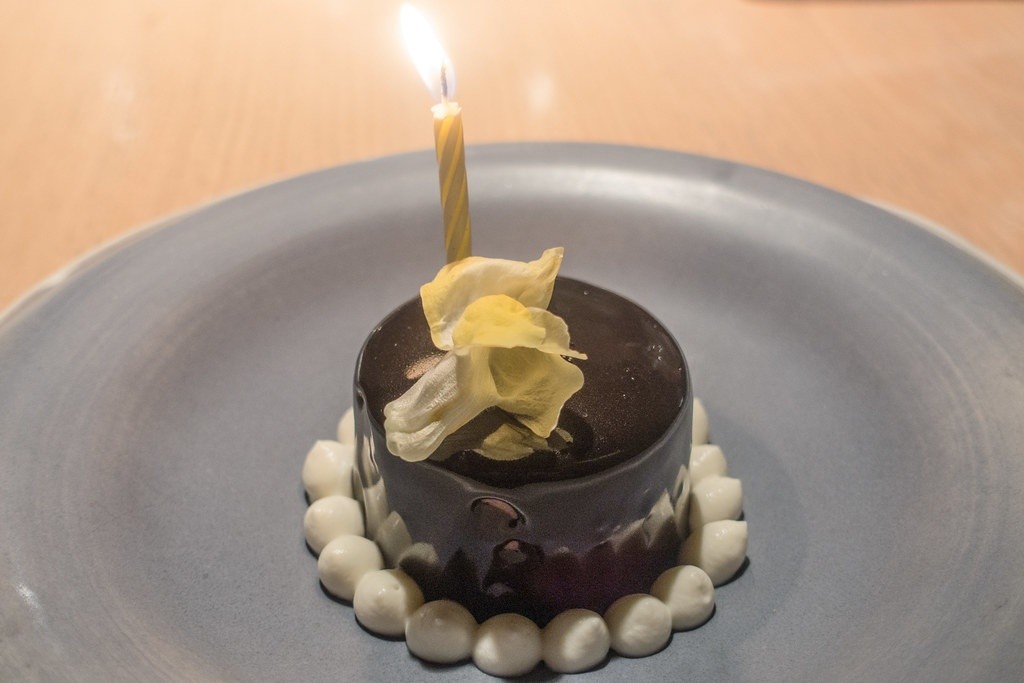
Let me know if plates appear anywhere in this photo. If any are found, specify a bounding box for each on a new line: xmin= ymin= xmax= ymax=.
xmin=0 ymin=140 xmax=1024 ymax=682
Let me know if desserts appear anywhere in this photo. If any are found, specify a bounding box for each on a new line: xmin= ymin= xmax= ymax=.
xmin=301 ymin=245 xmax=748 ymax=676
